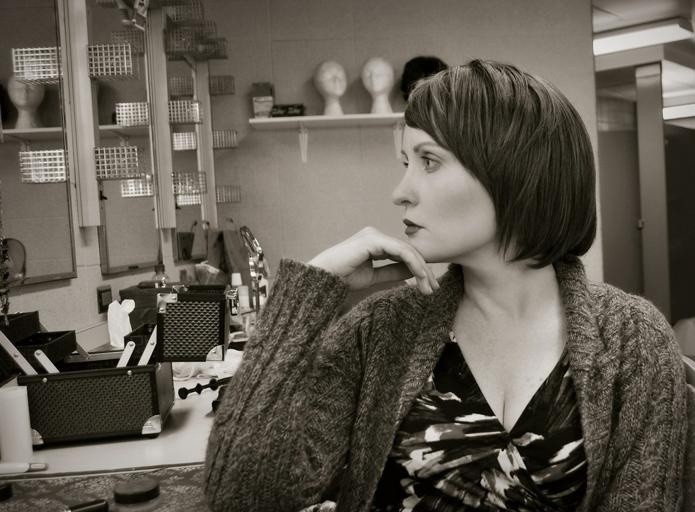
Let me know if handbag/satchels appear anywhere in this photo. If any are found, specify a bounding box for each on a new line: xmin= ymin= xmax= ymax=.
xmin=223 ymin=225 xmax=270 ymax=343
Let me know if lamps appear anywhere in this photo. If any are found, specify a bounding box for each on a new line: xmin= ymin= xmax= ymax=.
xmin=592 ymin=16 xmax=695 ymax=57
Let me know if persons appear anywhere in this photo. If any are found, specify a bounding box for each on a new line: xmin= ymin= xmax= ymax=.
xmin=314 ymin=58 xmax=346 ymax=116
xmin=363 ymin=56 xmax=394 ymax=115
xmin=7 ymin=69 xmax=47 ymax=128
xmin=199 ymin=62 xmax=695 ymax=512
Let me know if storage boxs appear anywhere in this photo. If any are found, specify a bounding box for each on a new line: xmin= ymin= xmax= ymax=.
xmin=18 ymin=348 xmax=175 ymax=449
xmin=156 ymin=284 xmax=229 ymax=363
